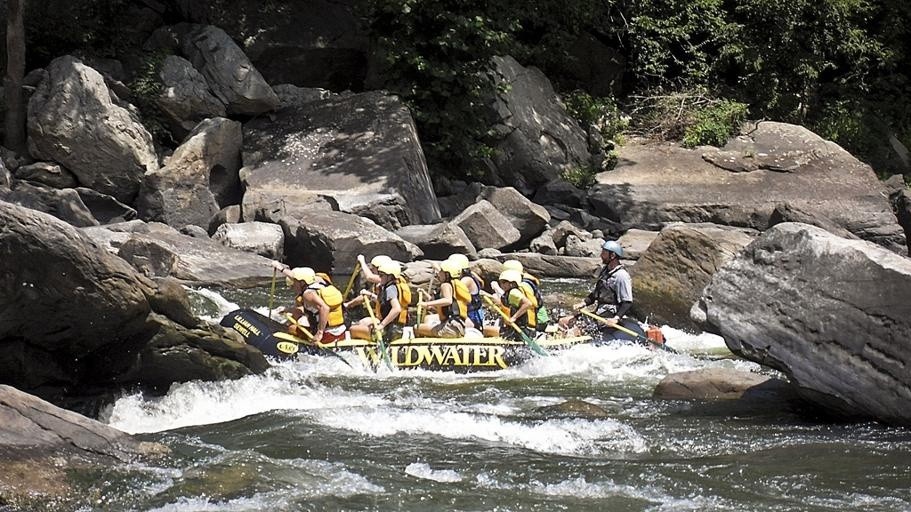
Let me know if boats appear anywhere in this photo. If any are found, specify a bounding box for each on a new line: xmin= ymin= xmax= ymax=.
xmin=220 ymin=307 xmax=665 ymax=372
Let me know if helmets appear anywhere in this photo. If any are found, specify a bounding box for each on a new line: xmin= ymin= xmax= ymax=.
xmin=499 ymin=270 xmax=521 ymax=287
xmin=440 ymin=260 xmax=462 ymax=278
xmin=448 ymin=254 xmax=469 ymax=269
xmin=503 ymin=260 xmax=523 ymax=272
xmin=601 ymin=241 xmax=622 ymax=257
xmin=371 ymin=255 xmax=392 ymax=268
xmin=378 ymin=260 xmax=401 ymax=279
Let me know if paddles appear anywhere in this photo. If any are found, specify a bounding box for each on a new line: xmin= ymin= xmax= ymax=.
xmin=363 ymin=294 xmax=397 ymax=373
xmin=578 ymin=310 xmax=674 ymax=352
xmin=284 ymin=313 xmax=351 ymax=366
xmin=483 ymin=296 xmax=548 ymax=358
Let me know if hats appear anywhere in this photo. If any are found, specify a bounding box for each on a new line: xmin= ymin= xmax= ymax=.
xmin=286 ymin=267 xmax=315 ymax=286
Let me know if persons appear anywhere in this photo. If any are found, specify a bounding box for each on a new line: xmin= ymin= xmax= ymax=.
xmin=559 ymin=240 xmax=633 ymax=335
xmin=271 ymin=255 xmax=550 ymax=346
